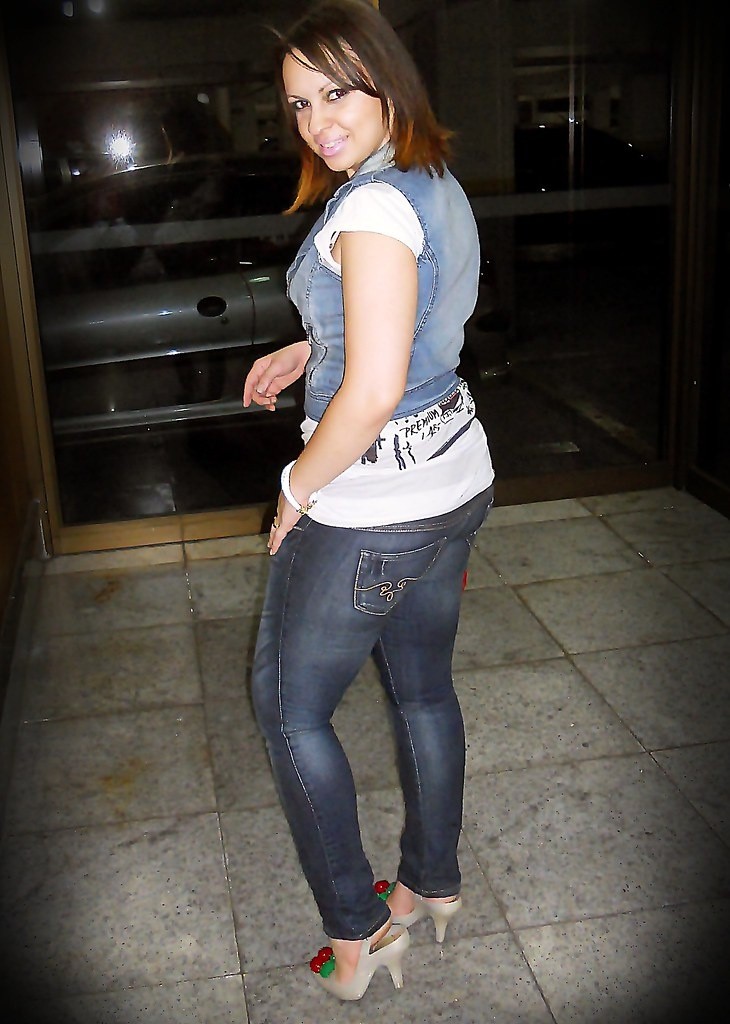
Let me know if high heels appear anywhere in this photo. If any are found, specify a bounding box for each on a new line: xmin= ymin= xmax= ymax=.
xmin=310 ymin=915 xmax=410 ymax=1000
xmin=373 ymin=880 xmax=462 ymax=943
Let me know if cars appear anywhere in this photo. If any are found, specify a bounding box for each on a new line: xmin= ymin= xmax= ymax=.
xmin=28 ymin=147 xmax=325 ymax=373
xmin=514 ymin=121 xmax=664 ymax=278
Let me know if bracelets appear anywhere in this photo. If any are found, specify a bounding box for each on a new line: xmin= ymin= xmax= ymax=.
xmin=280 ymin=459 xmax=317 ymax=514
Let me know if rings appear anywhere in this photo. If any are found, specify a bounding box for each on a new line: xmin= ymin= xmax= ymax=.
xmin=273 ymin=517 xmax=280 ymax=529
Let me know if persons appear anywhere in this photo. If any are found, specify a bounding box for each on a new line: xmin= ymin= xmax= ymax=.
xmin=244 ymin=0 xmax=499 ymax=1001
